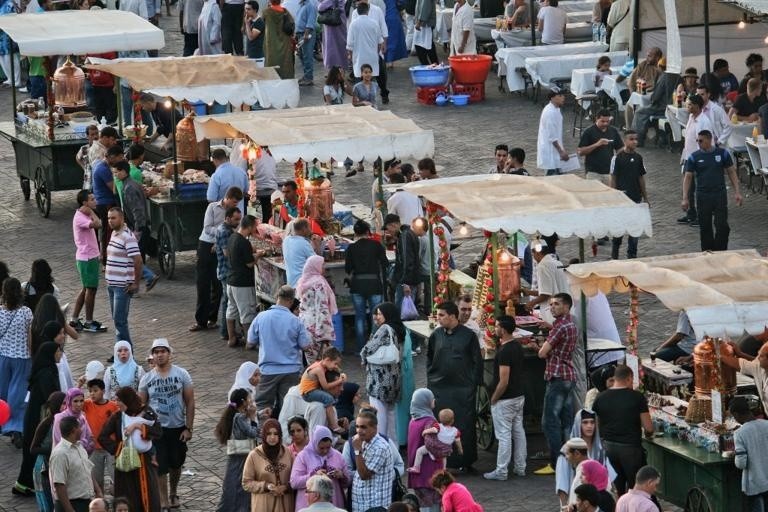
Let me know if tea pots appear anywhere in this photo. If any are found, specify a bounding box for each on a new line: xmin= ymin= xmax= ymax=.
xmin=434 ymin=92 xmax=450 ymax=106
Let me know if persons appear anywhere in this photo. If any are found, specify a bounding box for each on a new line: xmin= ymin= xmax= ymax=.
xmin=0 ymin=0 xmax=767 ymax=511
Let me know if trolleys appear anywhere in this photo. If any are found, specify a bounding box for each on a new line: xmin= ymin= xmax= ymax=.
xmin=240 ymin=203 xmax=387 ymax=319
xmin=0 ymin=114 xmax=116 ymax=217
xmin=398 ymin=305 xmax=627 ymax=450
xmin=120 ymin=138 xmax=232 ymax=279
xmin=561 ymin=362 xmax=768 ymax=512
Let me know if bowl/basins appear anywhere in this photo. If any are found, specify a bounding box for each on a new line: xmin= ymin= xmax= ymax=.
xmin=409 ymin=65 xmax=450 ymax=86
xmin=451 ymin=95 xmax=470 ymax=105
xmin=448 ymin=54 xmax=493 ymax=84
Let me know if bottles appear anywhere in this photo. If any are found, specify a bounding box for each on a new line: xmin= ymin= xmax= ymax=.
xmin=592 ymin=21 xmax=598 ymax=42
xmin=672 ymin=88 xmax=683 ymax=108
xmin=495 ymin=15 xmax=512 ymax=32
xmin=38 ymin=97 xmax=46 ymax=122
xmin=752 ymin=126 xmax=758 ymax=145
xmin=635 ymin=77 xmax=646 ymax=95
xmin=732 ymin=111 xmax=737 ymax=124
xmin=56 ymin=104 xmax=64 ymax=124
xmin=101 ymin=116 xmax=106 ymax=127
xmin=600 ymin=23 xmax=606 ymax=45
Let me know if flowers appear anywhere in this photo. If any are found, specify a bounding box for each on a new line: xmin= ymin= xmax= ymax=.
xmin=423 ymin=199 xmax=451 ymax=313
xmin=241 ymin=140 xmax=262 ymax=203
xmin=703 ymin=334 xmax=732 ymax=403
xmin=129 ymin=92 xmax=144 ymax=145
xmin=292 ymin=159 xmax=308 ymax=220
xmin=623 ymin=281 xmax=646 ymax=395
xmin=482 ymin=229 xmax=502 ymax=347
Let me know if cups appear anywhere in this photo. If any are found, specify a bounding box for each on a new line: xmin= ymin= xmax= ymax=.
xmin=19 ymin=102 xmax=35 ymax=115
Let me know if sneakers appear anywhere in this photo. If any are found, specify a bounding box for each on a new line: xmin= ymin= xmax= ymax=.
xmin=189 ymin=324 xmax=202 ymax=330
xmin=484 ymin=469 xmax=508 ymax=481
xmin=677 ymin=216 xmax=695 ymax=223
xmin=513 ymin=468 xmax=525 ymax=475
xmin=12 ymin=487 xmax=35 ymax=496
xmin=298 ymin=77 xmax=304 ymax=82
xmin=69 ymin=318 xmax=83 ymax=330
xmin=208 ymin=321 xmax=216 ymax=328
xmin=692 ymin=218 xmax=700 ymax=227
xmin=299 ymin=80 xmax=313 ymax=86
xmin=146 ymin=274 xmax=160 ymax=291
xmin=14 ymin=431 xmax=23 ymax=448
xmin=83 ymin=321 xmax=107 ymax=332
xmin=132 ymin=293 xmax=139 ymax=298
xmin=533 ymin=463 xmax=555 ymax=473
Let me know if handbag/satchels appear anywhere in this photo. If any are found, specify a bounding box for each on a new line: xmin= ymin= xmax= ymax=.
xmin=227 ymin=437 xmax=255 ymax=455
xmin=317 ymin=7 xmax=342 ymax=26
xmin=139 ymin=232 xmax=159 ymax=257
xmin=283 ymin=9 xmax=293 ymax=35
xmin=115 ymin=430 xmax=141 ymax=472
xmin=393 ymin=466 xmax=407 ymax=500
xmin=365 ymin=341 xmax=400 ymax=364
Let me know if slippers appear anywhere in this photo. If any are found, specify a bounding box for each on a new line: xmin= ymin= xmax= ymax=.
xmin=531 ymin=451 xmax=551 ymax=459
xmin=161 ymin=506 xmax=170 ymax=511
xmin=168 ymin=494 xmax=180 ymax=507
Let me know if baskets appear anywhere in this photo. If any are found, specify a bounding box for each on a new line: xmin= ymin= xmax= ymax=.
xmin=685 ymin=395 xmax=712 ymax=422
xmin=451 ymin=83 xmax=485 ymax=102
xmin=416 ymin=86 xmax=448 ymax=105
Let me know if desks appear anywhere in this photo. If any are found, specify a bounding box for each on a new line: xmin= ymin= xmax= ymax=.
xmin=436 ymin=0 xmax=768 ymax=199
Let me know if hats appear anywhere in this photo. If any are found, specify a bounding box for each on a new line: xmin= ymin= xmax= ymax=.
xmin=151 ymin=338 xmax=173 ymax=353
xmin=547 ymin=86 xmax=566 ymax=98
xmin=682 ymin=67 xmax=699 ymax=79
xmin=531 ymin=239 xmax=548 ymax=249
xmin=381 ymin=214 xmax=399 ymax=229
xmin=86 ymin=360 xmax=104 ymax=380
xmin=566 ymin=437 xmax=587 ymax=449
xmin=107 ymin=145 xmax=125 ymax=154
xmin=101 ymin=127 xmax=122 ymax=139
